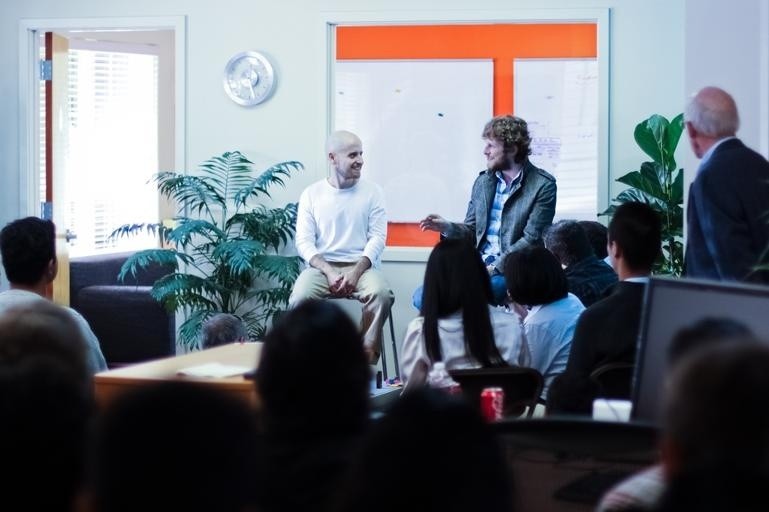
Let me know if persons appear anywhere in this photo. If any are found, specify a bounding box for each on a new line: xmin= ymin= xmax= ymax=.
xmin=589 ymin=337 xmax=769 ymax=512
xmin=564 ymin=200 xmax=664 ymax=376
xmin=287 ymin=129 xmax=395 ymax=366
xmin=595 ymin=313 xmax=760 ymax=512
xmin=540 ymin=220 xmax=620 ymax=306
xmin=503 ymin=246 xmax=589 ymax=404
xmin=400 ymin=235 xmax=531 ymax=389
xmin=411 ymin=116 xmax=556 ymax=316
xmin=1 ymin=294 xmax=522 ymax=511
xmin=1 ymin=217 xmax=115 ymax=376
xmin=680 ymin=86 xmax=769 ymax=288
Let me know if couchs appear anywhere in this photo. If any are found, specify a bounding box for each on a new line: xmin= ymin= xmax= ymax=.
xmin=68 ymin=251 xmax=177 ymax=361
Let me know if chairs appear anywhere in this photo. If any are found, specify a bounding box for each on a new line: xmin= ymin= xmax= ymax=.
xmin=580 ymin=362 xmax=633 ymax=414
xmin=449 ymin=366 xmax=546 ymax=421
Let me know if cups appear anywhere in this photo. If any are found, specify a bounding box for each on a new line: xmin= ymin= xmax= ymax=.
xmin=593 ymin=399 xmax=631 ymax=422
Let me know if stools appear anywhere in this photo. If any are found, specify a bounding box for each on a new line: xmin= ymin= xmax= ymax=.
xmin=306 ymin=290 xmax=399 ymax=382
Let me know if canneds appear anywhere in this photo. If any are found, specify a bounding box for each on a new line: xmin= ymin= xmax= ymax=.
xmin=479 ymin=386 xmax=506 ymax=422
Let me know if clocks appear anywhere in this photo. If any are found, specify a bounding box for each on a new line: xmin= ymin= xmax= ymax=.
xmin=220 ymin=50 xmax=276 ymax=107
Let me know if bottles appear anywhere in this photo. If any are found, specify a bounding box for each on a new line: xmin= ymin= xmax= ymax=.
xmin=425 ymin=362 xmax=453 ymax=392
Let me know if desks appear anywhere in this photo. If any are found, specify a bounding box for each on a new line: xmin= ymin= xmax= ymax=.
xmin=91 ymin=334 xmax=265 ymax=415
